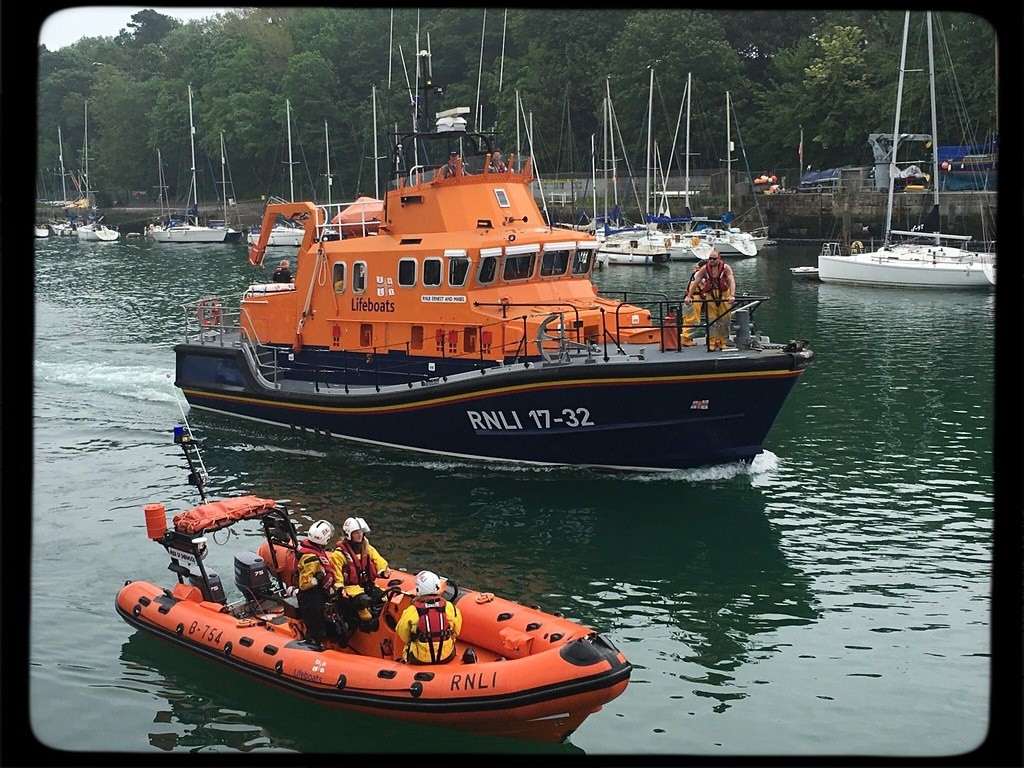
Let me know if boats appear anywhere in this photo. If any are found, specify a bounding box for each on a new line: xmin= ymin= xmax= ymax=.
xmin=174 ymin=9 xmax=817 ymax=479
xmin=114 ymin=382 xmax=634 ymax=746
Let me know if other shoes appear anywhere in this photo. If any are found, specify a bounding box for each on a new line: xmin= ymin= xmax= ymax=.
xmin=357 ymin=608 xmax=372 ymax=620
xmin=681 ymin=336 xmax=697 ymax=346
xmin=709 ymin=340 xmax=715 ymax=351
xmin=712 ymin=338 xmax=721 ymax=346
xmin=720 ymin=344 xmax=728 ymax=350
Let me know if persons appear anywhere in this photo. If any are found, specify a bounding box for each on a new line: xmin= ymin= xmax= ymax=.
xmin=684 ymin=251 xmax=735 ymax=351
xmin=681 ymin=260 xmax=707 ymax=347
xmin=395 ymin=571 xmax=462 ymax=665
xmin=297 ymin=520 xmax=355 ymax=651
xmin=273 ymin=260 xmax=296 ymax=283
xmin=489 ymin=149 xmax=514 ymax=174
xmin=329 ymin=515 xmax=392 ymax=630
xmin=441 ymin=151 xmax=472 ymax=179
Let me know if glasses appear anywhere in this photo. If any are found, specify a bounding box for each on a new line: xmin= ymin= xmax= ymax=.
xmin=709 ymin=257 xmax=719 ymax=260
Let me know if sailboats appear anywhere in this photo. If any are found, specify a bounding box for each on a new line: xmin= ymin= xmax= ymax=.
xmin=32 ymin=99 xmax=121 ymax=242
xmin=125 ymin=79 xmax=244 ymax=245
xmin=248 ymin=7 xmax=998 ymax=293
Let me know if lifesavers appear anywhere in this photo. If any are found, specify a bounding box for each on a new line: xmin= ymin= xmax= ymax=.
xmin=692 ymin=237 xmax=698 ymax=246
xmin=196 ymin=299 xmax=220 ymax=326
xmin=852 ymin=240 xmax=863 ymax=253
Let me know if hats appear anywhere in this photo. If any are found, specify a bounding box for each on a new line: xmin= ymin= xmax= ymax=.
xmin=698 ymin=260 xmax=707 ymax=267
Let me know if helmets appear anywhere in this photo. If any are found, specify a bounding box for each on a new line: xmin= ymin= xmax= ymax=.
xmin=416 ymin=570 xmax=439 ymax=595
xmin=308 ymin=520 xmax=335 ymax=546
xmin=342 ymin=517 xmax=366 ymax=540
